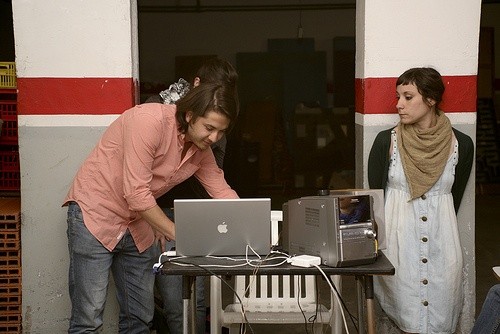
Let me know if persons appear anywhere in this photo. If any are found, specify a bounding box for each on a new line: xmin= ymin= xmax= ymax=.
xmin=339 ymin=197 xmax=370 ymax=225
xmin=367 ymin=67 xmax=474 ymax=334
xmin=60 ymin=79 xmax=241 ymax=334
xmin=143 ymin=57 xmax=239 ymax=334
xmin=471 ymin=283 xmax=500 ymax=334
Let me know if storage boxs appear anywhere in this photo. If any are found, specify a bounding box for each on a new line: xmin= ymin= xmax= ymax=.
xmin=0 ymin=60 xmax=21 ymax=191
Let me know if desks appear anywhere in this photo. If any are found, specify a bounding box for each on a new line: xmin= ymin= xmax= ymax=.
xmin=162 ymin=246 xmax=395 ymax=334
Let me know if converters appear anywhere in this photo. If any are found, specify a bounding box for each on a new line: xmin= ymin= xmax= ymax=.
xmin=291 ymin=255 xmax=321 ymax=267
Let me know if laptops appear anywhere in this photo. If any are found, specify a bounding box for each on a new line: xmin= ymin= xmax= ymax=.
xmin=173 ymin=198 xmax=272 ymax=256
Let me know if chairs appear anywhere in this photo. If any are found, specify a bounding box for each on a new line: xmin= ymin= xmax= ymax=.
xmin=210 ymin=210 xmax=343 ymax=334
